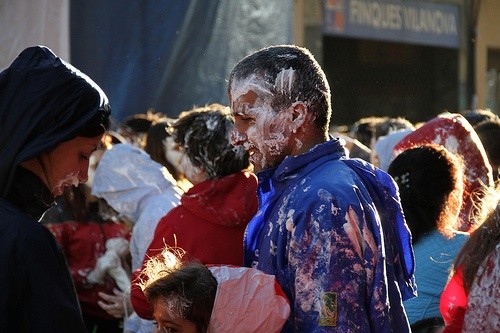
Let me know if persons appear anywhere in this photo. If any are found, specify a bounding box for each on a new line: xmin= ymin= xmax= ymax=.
xmin=327 ymin=106 xmax=500 ymax=333
xmin=0 ymin=44 xmax=112 ymax=333
xmin=221 ymin=45 xmax=419 ymax=333
xmin=39 ymin=98 xmax=264 ymax=333
xmin=144 ymin=263 xmax=219 ymax=333
